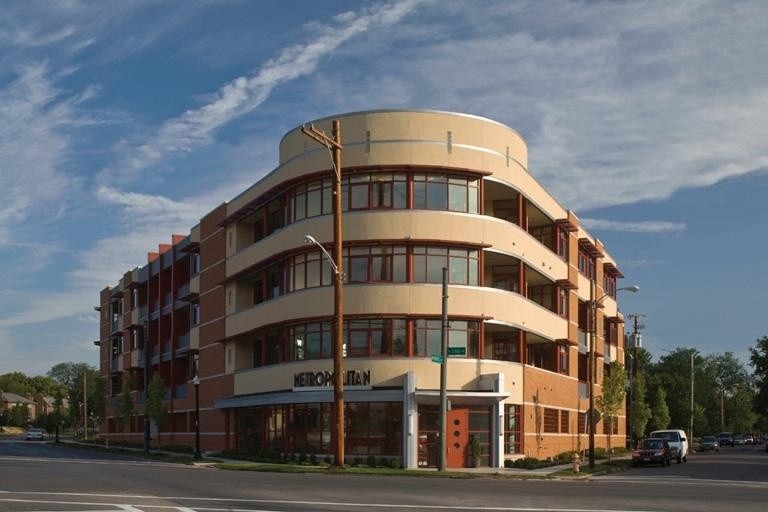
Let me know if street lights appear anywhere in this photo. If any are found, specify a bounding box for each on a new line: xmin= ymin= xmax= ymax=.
xmin=191 ymin=375 xmax=201 ymax=462
xmin=301 ymin=231 xmax=347 ymax=464
xmin=587 ymin=280 xmax=640 ymax=471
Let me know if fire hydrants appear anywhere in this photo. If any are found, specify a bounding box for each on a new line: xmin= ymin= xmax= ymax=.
xmin=103 ymin=439 xmax=112 ymax=451
xmin=568 ymin=453 xmax=583 ymax=474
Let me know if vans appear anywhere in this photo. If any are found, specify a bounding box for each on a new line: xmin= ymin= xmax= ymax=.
xmin=650 ymin=430 xmax=689 ymax=464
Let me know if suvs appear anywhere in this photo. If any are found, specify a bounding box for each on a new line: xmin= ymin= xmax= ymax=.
xmin=629 ymin=438 xmax=672 ymax=467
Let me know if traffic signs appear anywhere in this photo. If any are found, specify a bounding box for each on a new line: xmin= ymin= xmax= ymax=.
xmin=431 ymin=353 xmax=444 ymax=364
xmin=447 ymin=346 xmax=467 ymax=355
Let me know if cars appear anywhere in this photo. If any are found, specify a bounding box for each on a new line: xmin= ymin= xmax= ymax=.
xmin=76 ymin=427 xmax=85 ymax=435
xmin=26 ymin=428 xmax=47 ymax=440
xmin=697 ymin=430 xmax=767 ymax=453
